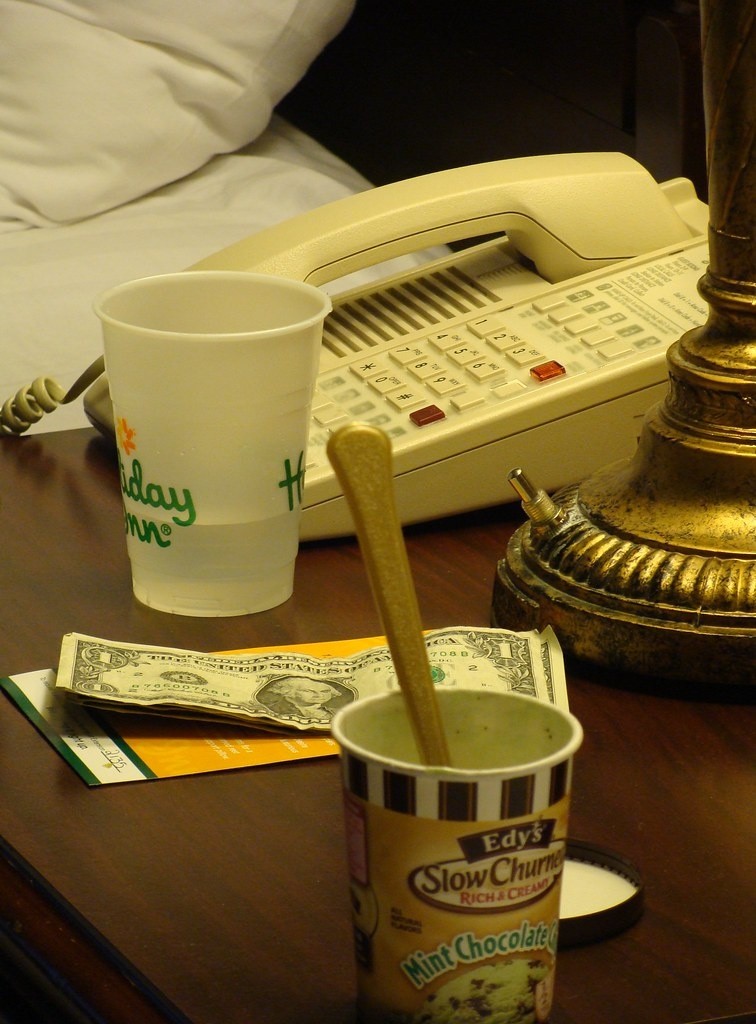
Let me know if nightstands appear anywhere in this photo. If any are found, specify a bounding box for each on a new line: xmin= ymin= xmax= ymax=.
xmin=0 ymin=427 xmax=756 ymax=1024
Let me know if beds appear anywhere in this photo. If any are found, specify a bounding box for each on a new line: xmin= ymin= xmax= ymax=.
xmin=0 ymin=1 xmax=703 ymax=435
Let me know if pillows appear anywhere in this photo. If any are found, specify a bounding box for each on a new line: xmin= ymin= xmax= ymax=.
xmin=0 ymin=0 xmax=355 ymax=237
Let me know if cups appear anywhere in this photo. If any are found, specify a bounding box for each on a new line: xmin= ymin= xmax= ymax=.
xmin=93 ymin=271 xmax=331 ymax=617
xmin=331 ymin=686 xmax=583 ymax=1023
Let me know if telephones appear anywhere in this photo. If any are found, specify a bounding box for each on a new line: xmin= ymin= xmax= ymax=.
xmin=78 ymin=148 xmax=718 ymax=544
